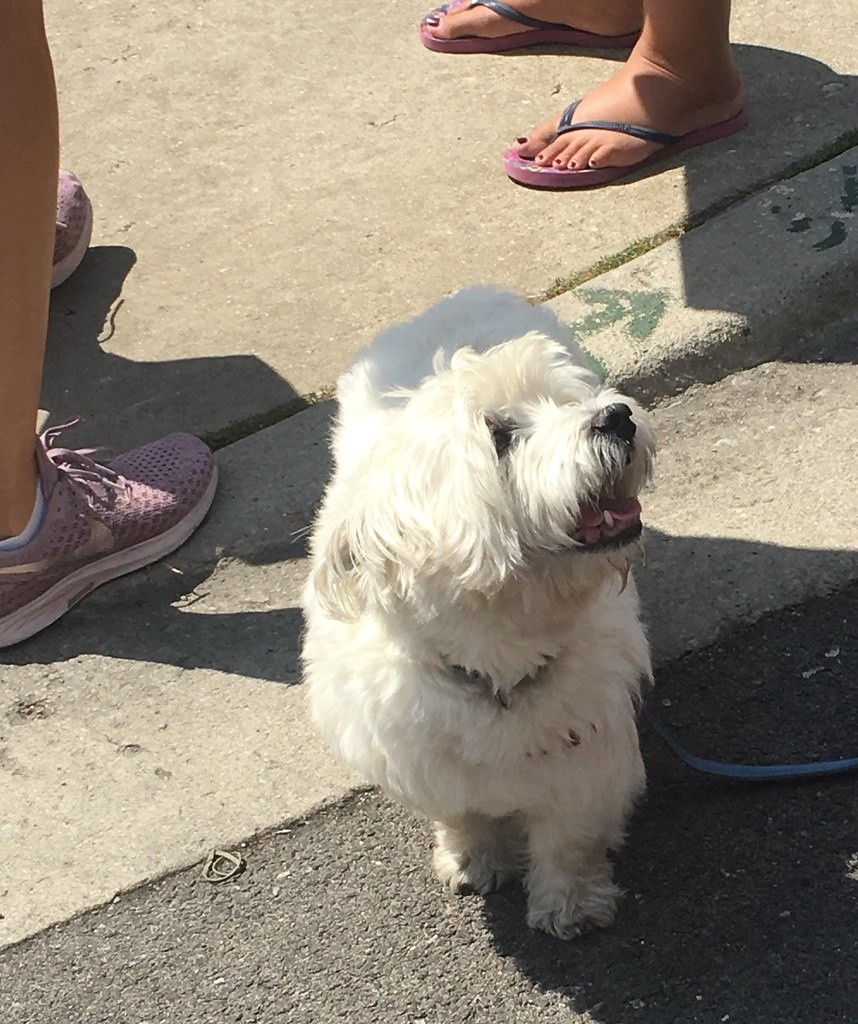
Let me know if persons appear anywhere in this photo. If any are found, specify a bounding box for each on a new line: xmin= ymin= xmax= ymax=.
xmin=0 ymin=0 xmax=216 ymax=650
xmin=421 ymin=1 xmax=748 ymax=186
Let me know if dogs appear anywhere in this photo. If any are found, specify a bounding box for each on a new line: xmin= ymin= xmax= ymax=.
xmin=294 ymin=282 xmax=660 ymax=938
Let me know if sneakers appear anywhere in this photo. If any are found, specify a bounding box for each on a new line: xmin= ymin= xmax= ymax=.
xmin=47 ymin=166 xmax=94 ymax=290
xmin=0 ymin=408 xmax=220 ymax=649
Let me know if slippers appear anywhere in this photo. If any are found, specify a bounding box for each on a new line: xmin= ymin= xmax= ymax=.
xmin=418 ymin=0 xmax=644 ymax=54
xmin=502 ymin=64 xmax=749 ymax=191
